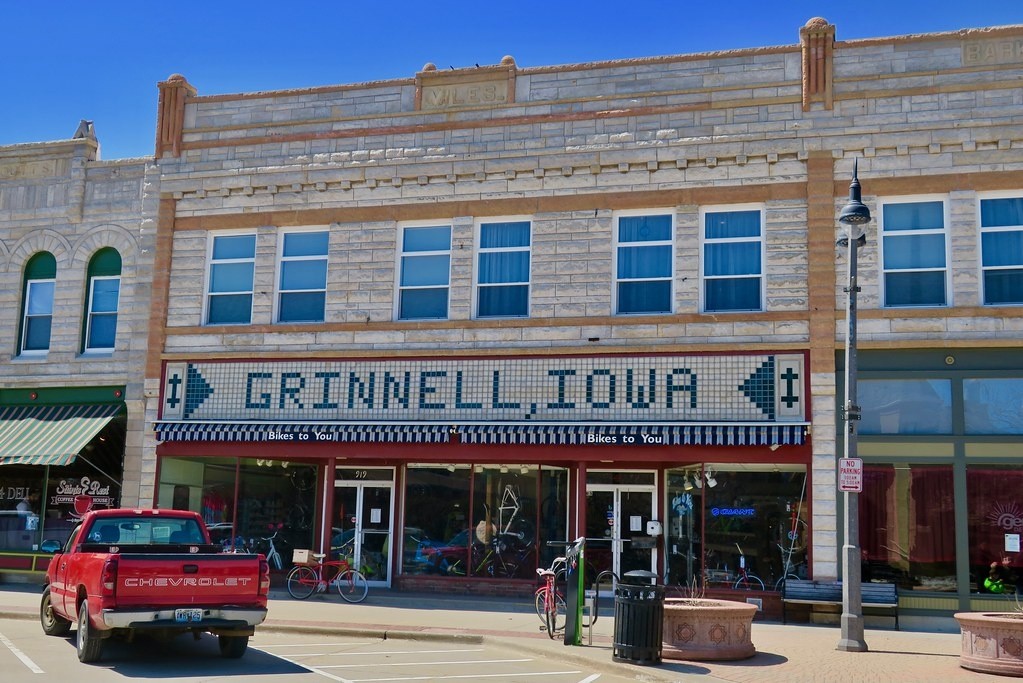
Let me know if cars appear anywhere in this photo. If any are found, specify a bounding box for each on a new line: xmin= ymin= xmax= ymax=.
xmin=330 ymin=527 xmax=441 ymax=577
xmin=419 ymin=525 xmax=483 ymax=567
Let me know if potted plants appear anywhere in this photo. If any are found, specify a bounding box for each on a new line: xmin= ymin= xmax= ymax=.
xmin=661 ymin=576 xmax=759 ymax=660
xmin=954 ymin=591 xmax=1023 ymax=673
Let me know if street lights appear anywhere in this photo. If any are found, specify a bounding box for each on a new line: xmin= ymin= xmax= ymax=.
xmin=830 ymin=154 xmax=869 ymax=651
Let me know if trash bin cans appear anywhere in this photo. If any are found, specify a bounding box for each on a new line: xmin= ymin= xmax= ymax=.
xmin=611 ymin=570 xmax=666 ymax=666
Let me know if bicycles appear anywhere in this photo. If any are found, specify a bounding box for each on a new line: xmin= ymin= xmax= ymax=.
xmin=287 ymin=536 xmax=370 ymax=604
xmin=446 ymin=484 xmax=536 ymax=581
xmin=230 ymin=532 xmax=284 ymax=571
xmin=730 ymin=542 xmax=767 ymax=592
xmin=287 ymin=464 xmax=317 ymax=530
xmin=773 ymin=495 xmax=809 ymax=594
xmin=535 ymin=557 xmax=567 ymax=639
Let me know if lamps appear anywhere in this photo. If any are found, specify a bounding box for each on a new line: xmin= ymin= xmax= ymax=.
xmin=282 ymin=461 xmax=289 ymax=468
xmin=266 ymin=460 xmax=272 ymax=467
xmin=500 ymin=464 xmax=508 ymax=473
xmin=693 ymin=468 xmax=702 ymax=489
xmin=704 ymin=465 xmax=716 ymax=489
xmin=474 ymin=464 xmax=483 ymax=473
xmin=683 ymin=470 xmax=693 ymax=490
xmin=256 ymin=459 xmax=265 ymax=466
xmin=520 ymin=464 xmax=529 ymax=474
xmin=447 ymin=463 xmax=457 ymax=473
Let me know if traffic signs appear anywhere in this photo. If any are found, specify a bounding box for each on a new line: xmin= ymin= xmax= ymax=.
xmin=837 ymin=458 xmax=864 ymax=493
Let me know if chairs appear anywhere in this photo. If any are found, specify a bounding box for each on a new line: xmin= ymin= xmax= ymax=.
xmin=98 ymin=525 xmax=120 ymax=544
xmin=169 ymin=531 xmax=192 ymax=543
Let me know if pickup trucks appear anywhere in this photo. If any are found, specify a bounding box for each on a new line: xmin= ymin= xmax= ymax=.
xmin=39 ymin=508 xmax=270 ymax=664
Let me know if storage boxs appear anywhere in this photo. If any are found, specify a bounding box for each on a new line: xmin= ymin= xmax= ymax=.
xmin=293 ymin=549 xmax=315 ymax=563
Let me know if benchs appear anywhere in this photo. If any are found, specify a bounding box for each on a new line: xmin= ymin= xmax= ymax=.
xmin=780 ymin=580 xmax=900 ymax=631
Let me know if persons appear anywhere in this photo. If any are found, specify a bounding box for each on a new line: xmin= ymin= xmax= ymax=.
xmin=17 ymin=484 xmax=40 ymax=517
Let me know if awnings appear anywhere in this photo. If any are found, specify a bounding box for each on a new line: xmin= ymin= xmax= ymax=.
xmin=0 ymin=404 xmax=126 ymax=486
